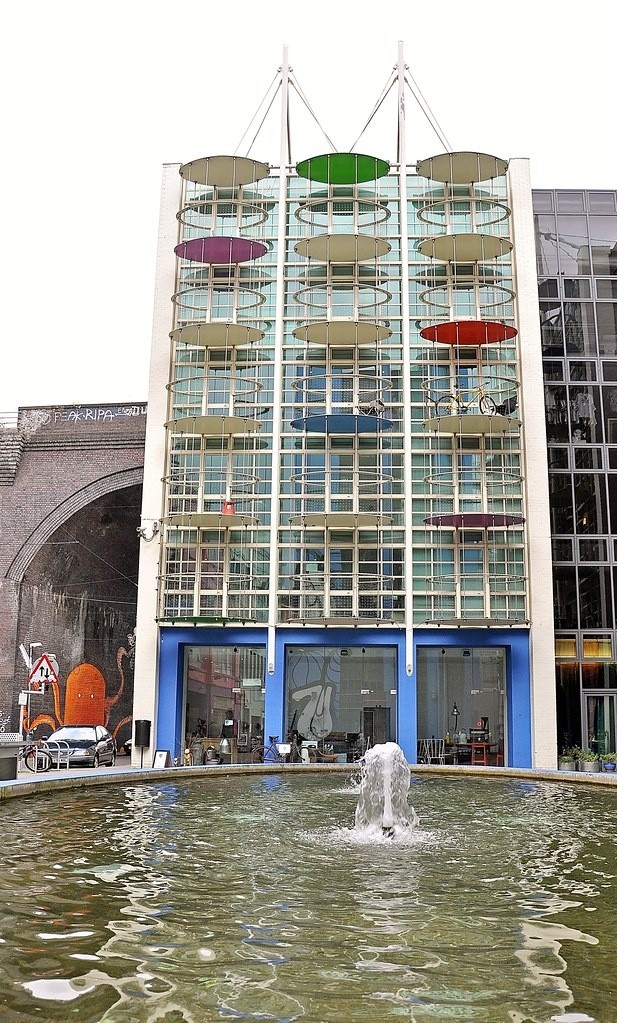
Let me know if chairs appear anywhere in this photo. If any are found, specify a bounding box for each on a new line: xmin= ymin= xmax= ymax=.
xmin=496 ymin=754 xmax=503 ymax=767
xmin=417 ymin=739 xmax=451 ymax=765
xmin=472 ymin=742 xmax=486 ymax=766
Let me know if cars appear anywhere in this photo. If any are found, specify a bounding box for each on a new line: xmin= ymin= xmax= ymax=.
xmin=36 ymin=724 xmax=117 ymax=771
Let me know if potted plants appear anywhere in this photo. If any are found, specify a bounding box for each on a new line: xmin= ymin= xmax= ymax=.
xmin=559 ymin=744 xmax=617 ymax=772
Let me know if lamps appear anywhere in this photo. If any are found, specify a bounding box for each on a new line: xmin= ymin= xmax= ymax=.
xmin=451 ymin=702 xmax=460 ymax=732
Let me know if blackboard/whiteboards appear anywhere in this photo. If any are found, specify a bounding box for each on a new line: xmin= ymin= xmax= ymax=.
xmin=152 ymin=750 xmax=169 ymax=769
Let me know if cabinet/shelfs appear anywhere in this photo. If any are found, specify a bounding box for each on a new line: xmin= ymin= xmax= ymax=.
xmin=197 ymin=739 xmax=237 ymax=764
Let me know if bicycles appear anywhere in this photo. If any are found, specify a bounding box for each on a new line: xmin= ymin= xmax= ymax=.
xmin=249 ymin=728 xmax=317 ymax=764
xmin=18 ymin=731 xmax=54 ymax=773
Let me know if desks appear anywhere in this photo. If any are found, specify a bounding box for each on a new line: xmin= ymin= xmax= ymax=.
xmin=456 ymin=743 xmax=497 ymax=755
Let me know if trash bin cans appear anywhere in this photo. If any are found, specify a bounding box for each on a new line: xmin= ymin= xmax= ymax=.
xmin=0 ymin=757 xmax=18 ymax=780
xmin=134 ymin=719 xmax=152 ymax=747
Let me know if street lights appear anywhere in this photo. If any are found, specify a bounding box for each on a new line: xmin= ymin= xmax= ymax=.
xmin=27 ymin=642 xmax=43 ymax=734
